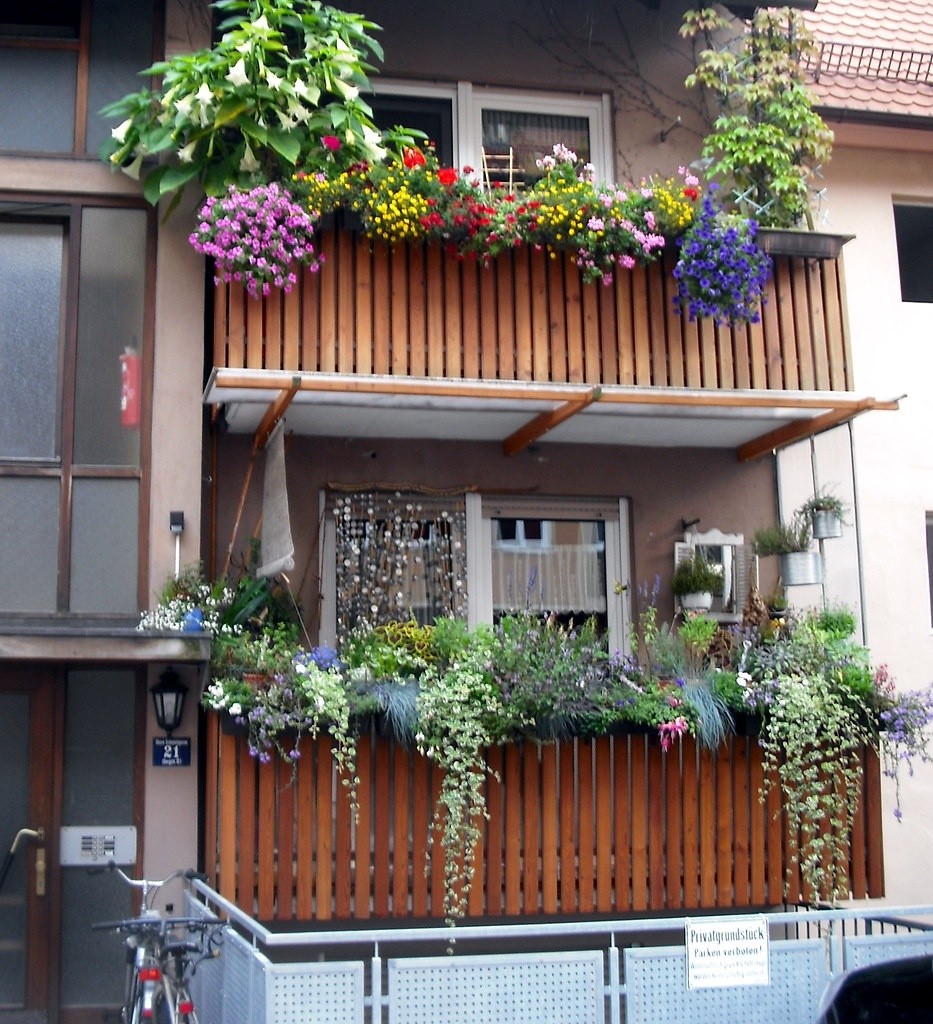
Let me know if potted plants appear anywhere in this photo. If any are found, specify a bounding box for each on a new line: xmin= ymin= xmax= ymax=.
xmin=675 ymin=559 xmax=722 ymax=615
xmin=800 ymin=490 xmax=853 ymax=538
xmin=679 ymin=3 xmax=858 ymax=258
xmin=756 ymin=522 xmax=819 ymax=584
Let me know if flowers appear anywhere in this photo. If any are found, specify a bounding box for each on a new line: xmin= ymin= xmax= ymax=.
xmin=188 ymin=135 xmax=778 ymax=328
xmin=211 ymin=547 xmax=933 ymax=962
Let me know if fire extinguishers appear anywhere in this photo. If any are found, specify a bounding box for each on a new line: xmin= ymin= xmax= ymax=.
xmin=119 ymin=333 xmax=141 ymax=428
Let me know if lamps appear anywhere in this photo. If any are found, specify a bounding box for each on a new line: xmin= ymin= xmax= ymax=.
xmin=150 ymin=665 xmax=190 ymax=739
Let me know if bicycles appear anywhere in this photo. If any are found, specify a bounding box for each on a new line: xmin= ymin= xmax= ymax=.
xmin=86 ymin=860 xmax=234 ymax=1024
